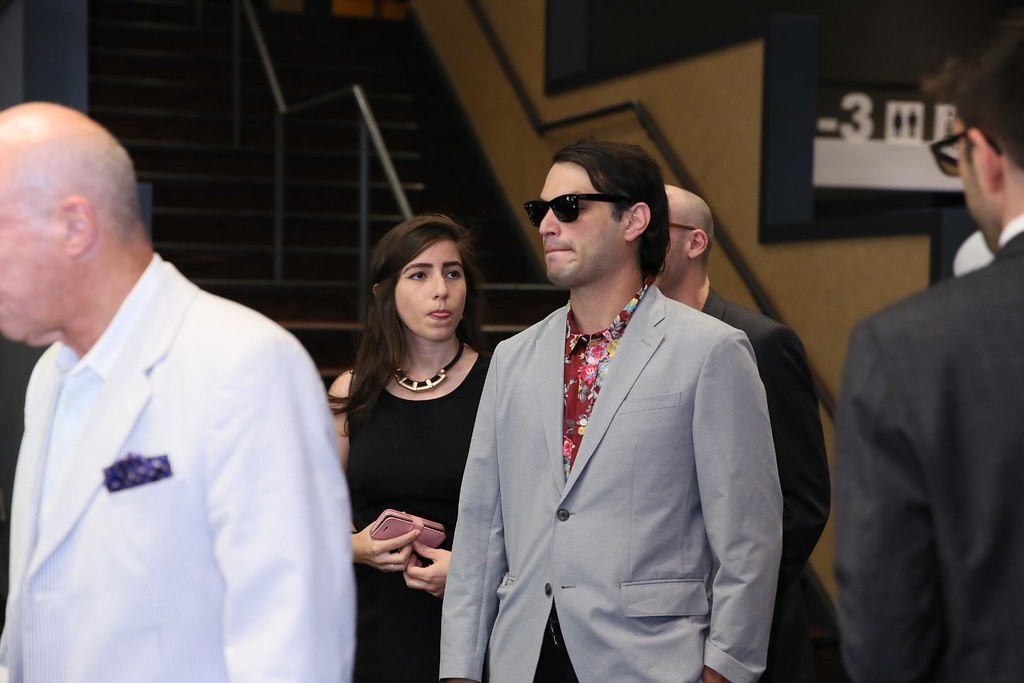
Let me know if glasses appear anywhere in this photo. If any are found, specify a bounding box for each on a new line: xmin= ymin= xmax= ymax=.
xmin=930 ymin=129 xmax=1002 ymax=176
xmin=523 ymin=192 xmax=625 ymax=227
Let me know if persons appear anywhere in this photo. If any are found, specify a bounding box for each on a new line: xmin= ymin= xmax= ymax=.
xmin=327 ymin=213 xmax=492 ymax=683
xmin=439 ymin=136 xmax=785 ymax=683
xmin=653 ymin=183 xmax=832 ymax=683
xmin=832 ymin=21 xmax=1024 ymax=683
xmin=0 ymin=101 xmax=356 ymax=683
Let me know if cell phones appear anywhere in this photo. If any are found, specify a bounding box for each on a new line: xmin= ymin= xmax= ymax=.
xmin=368 ymin=511 xmax=445 ymax=549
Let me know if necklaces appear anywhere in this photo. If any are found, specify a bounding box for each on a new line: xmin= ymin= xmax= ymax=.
xmin=395 ymin=335 xmax=464 ymax=393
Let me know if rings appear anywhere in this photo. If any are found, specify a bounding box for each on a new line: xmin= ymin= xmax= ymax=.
xmin=391 ymin=563 xmax=394 ymax=572
